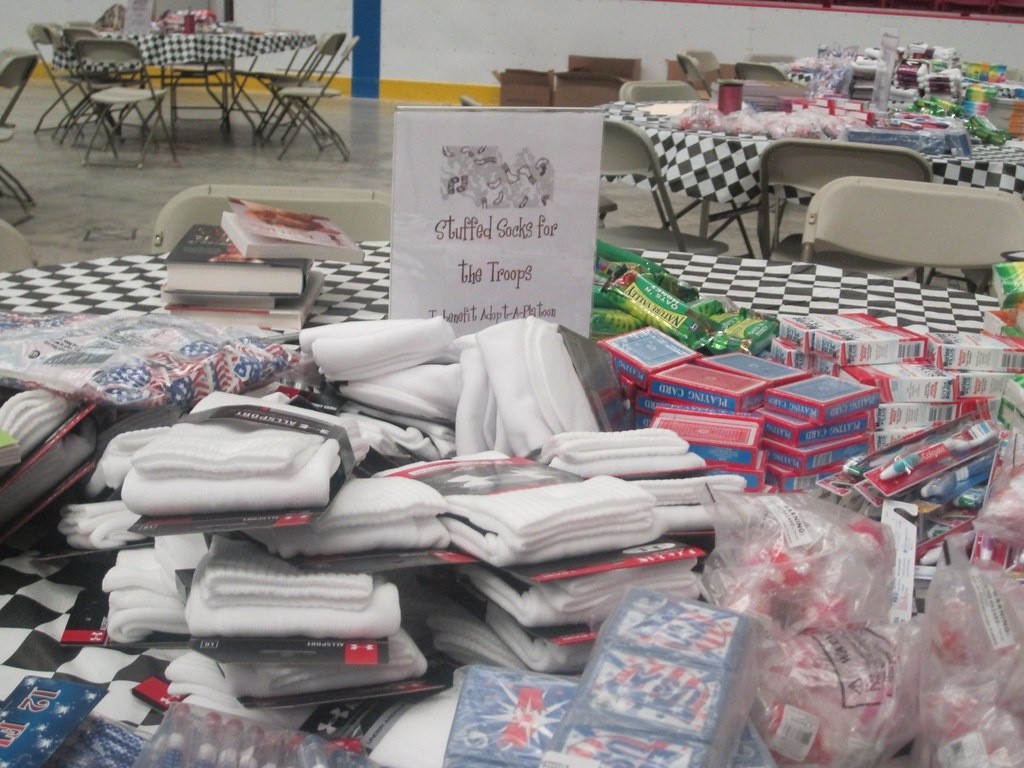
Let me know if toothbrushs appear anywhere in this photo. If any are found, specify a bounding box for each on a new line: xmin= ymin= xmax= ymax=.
xmin=815 ymin=417 xmax=1024 ymax=577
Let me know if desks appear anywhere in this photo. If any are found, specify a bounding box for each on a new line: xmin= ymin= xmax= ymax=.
xmin=588 ymin=102 xmax=1024 ymax=261
xmin=50 ymin=26 xmax=317 ymax=143
xmin=0 ymin=256 xmax=1024 ymax=739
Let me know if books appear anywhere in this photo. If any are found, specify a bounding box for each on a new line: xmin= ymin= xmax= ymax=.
xmin=160 ymin=196 xmax=367 ymax=340
xmin=709 ymin=80 xmax=810 ymax=112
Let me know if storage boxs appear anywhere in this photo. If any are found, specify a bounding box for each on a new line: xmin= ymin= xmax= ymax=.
xmin=568 ymin=54 xmax=641 ymax=83
xmin=666 ymin=59 xmax=737 ymax=101
xmin=444 ymin=312 xmax=1024 ymax=767
xmin=553 ymin=70 xmax=633 ymax=107
xmin=490 ymin=65 xmax=554 ymax=108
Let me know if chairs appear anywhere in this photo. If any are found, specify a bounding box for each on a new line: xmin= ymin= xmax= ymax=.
xmin=151 ymin=184 xmax=393 ymax=258
xmin=0 ymin=53 xmax=40 ymax=215
xmin=28 ymin=22 xmax=361 ymax=161
xmin=462 ymin=48 xmax=1023 ymax=297
